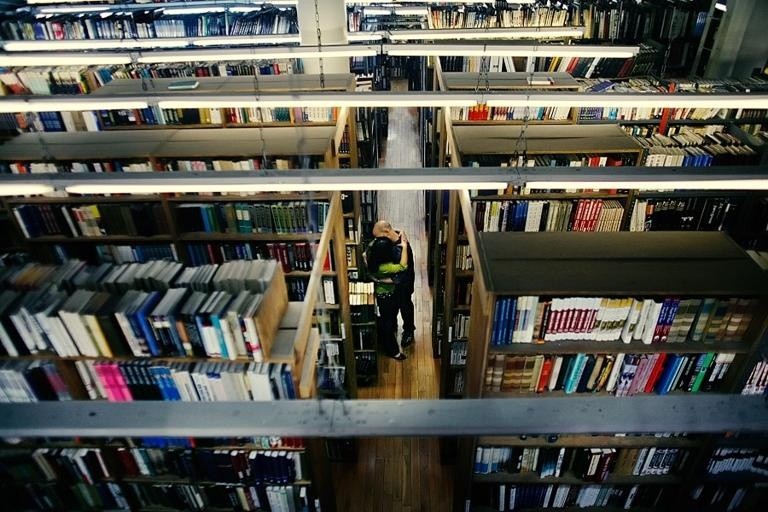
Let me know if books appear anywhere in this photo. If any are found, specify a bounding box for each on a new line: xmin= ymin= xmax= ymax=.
xmin=0 ymin=59 xmax=307 ymax=136
xmin=1 ymin=259 xmax=321 ymax=511
xmin=346 ymin=1 xmax=624 ymax=78
xmin=436 ymin=220 xmax=474 ymax=394
xmin=0 ymin=157 xmax=333 ymax=273
xmin=1 ymin=9 xmax=298 ymax=41
xmin=440 ymin=49 xmax=768 ymax=96
xmin=466 ymin=296 xmax=768 ymax=512
xmin=309 ymin=83 xmax=378 ymax=378
xmin=437 ymin=105 xmax=768 ymax=232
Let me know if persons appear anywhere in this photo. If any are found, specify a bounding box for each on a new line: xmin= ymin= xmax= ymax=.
xmin=363 ymin=221 xmax=415 ymax=359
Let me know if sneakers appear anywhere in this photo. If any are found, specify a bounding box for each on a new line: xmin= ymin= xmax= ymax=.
xmin=401 ymin=334 xmax=413 ymax=347
xmin=393 ymin=353 xmax=406 ymax=360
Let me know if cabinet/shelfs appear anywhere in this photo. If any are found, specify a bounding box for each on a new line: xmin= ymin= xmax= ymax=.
xmin=0 ymin=0 xmax=768 ymax=512
